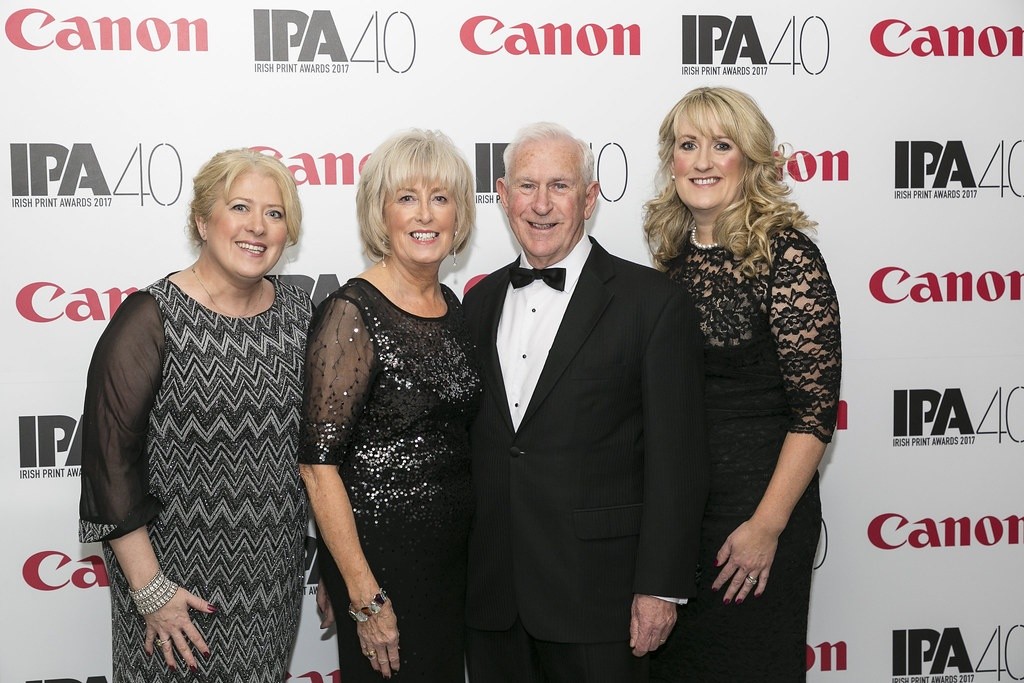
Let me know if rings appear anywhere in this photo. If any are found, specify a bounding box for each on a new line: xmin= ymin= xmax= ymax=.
xmin=156 ymin=638 xmax=170 ymax=646
xmin=746 ymin=576 xmax=757 ymax=585
xmin=366 ymin=650 xmax=376 ymax=655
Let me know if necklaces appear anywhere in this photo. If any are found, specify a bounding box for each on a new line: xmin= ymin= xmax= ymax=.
xmin=690 ymin=226 xmax=718 ymax=250
xmin=192 ymin=264 xmax=263 ymax=317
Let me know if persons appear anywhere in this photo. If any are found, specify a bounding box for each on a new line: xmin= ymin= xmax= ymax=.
xmin=78 ymin=148 xmax=335 ymax=683
xmin=461 ymin=122 xmax=710 ymax=683
xmin=298 ymin=129 xmax=476 ymax=683
xmin=644 ymin=87 xmax=842 ymax=683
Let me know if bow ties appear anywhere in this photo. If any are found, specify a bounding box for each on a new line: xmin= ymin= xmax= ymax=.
xmin=508 ymin=267 xmax=566 ymax=291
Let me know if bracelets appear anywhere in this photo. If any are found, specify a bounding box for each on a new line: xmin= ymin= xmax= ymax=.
xmin=348 ymin=588 xmax=387 ymax=622
xmin=128 ymin=567 xmax=178 ymax=615
